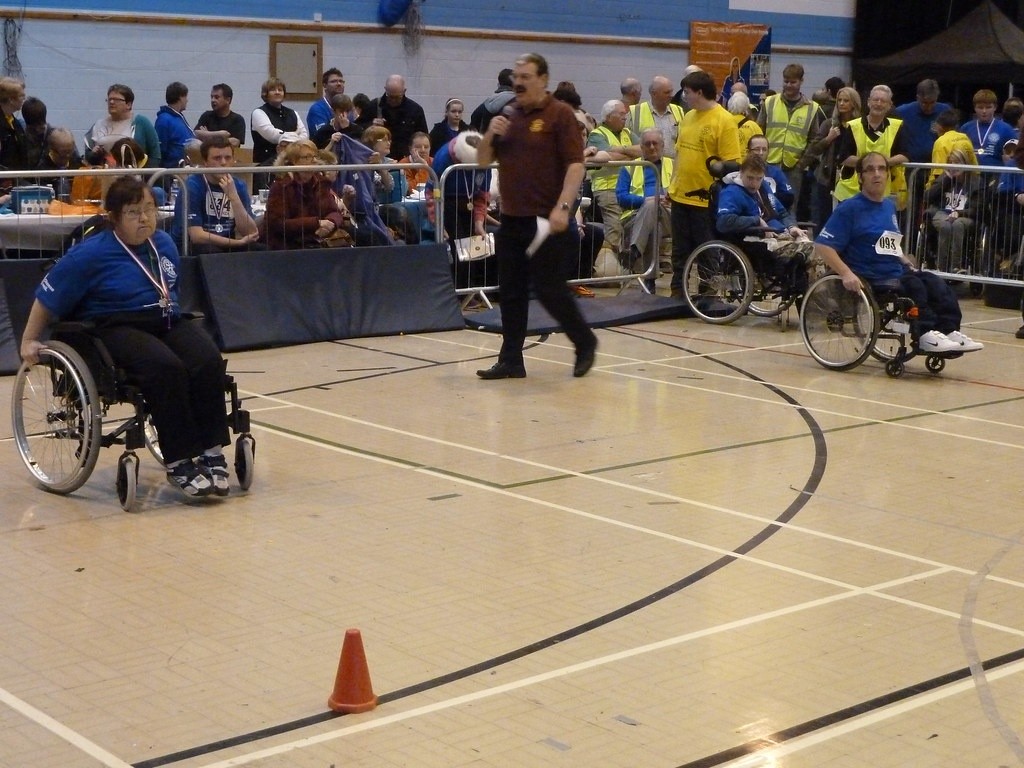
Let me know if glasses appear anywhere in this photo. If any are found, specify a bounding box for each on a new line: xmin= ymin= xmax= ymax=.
xmin=121 ymin=209 xmax=155 ymax=218
xmin=750 ymin=148 xmax=768 ymax=152
xmin=106 ymin=97 xmax=126 ymax=103
xmin=509 ymin=74 xmax=535 ymax=83
xmin=861 ymin=166 xmax=887 ymax=173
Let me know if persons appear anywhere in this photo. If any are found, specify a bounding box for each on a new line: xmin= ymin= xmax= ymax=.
xmin=250 ymin=68 xmax=525 ymax=309
xmin=0 ymin=76 xmax=162 ymax=209
xmin=154 ymin=81 xmax=247 ymax=176
xmin=20 ymin=174 xmax=231 ymax=498
xmin=168 ymin=134 xmax=260 ymax=257
xmin=813 ymin=151 xmax=986 ymax=354
xmin=549 ymin=63 xmax=1024 ymax=338
xmin=720 ymin=56 xmax=747 ymax=111
xmin=750 ymin=55 xmax=769 ymax=84
xmin=476 ymin=53 xmax=599 ymax=380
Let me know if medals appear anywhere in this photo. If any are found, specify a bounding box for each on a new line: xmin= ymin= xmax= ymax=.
xmin=951 ymin=210 xmax=959 ymax=218
xmin=215 ymin=223 xmax=224 ymax=234
xmin=158 ymin=298 xmax=168 ymax=308
xmin=466 ymin=201 xmax=475 ymax=211
xmin=977 ymin=148 xmax=985 ymax=155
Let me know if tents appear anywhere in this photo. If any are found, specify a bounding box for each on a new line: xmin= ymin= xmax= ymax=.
xmin=856 ymin=0 xmax=1024 ymax=91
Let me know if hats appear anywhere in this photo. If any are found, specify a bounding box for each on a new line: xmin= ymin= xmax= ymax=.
xmin=1003 ymin=139 xmax=1019 ymax=147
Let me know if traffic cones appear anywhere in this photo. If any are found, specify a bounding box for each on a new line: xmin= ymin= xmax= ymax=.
xmin=325 ymin=626 xmax=380 ymax=714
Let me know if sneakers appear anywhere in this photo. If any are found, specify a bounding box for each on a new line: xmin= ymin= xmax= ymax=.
xmin=476 ymin=361 xmax=526 ymax=378
xmin=167 ymin=453 xmax=230 ymax=500
xmin=573 ymin=336 xmax=597 ymax=377
xmin=919 ymin=330 xmax=984 ymax=351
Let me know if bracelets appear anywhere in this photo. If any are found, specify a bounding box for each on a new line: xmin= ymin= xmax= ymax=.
xmin=558 ymin=200 xmax=572 ymax=210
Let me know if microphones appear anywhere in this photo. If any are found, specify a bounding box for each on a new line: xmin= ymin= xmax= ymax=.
xmin=491 ymin=106 xmax=514 ymax=147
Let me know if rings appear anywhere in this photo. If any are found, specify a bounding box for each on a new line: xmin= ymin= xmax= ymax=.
xmin=593 ymin=148 xmax=594 ymax=150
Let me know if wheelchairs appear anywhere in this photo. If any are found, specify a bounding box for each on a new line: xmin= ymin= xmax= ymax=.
xmin=798 ymin=266 xmax=967 ymax=378
xmin=682 ymin=156 xmax=818 ymax=324
xmin=915 ymin=173 xmax=995 ymax=297
xmin=12 ymin=254 xmax=259 ymax=511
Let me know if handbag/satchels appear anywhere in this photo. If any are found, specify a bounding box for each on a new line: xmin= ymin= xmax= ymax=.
xmin=814 ymin=157 xmax=833 ymax=186
xmin=99 ymin=143 xmax=137 ymax=208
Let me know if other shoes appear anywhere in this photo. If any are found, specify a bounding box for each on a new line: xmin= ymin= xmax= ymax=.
xmin=571 ymin=285 xmax=595 ymax=298
xmin=641 ymin=279 xmax=656 ymax=294
xmin=621 ymin=248 xmax=637 ymax=269
xmin=1016 ymin=325 xmax=1024 ymax=338
xmin=660 ymin=260 xmax=673 ymax=272
xmin=698 ymin=285 xmax=718 ymax=295
xmin=670 ymin=288 xmax=684 ymax=299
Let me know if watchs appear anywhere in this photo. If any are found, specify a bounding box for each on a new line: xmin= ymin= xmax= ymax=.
xmin=622 ymin=144 xmax=627 ymax=154
